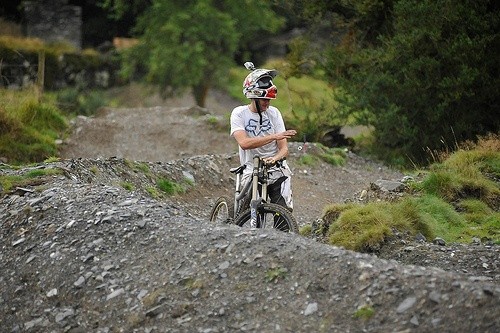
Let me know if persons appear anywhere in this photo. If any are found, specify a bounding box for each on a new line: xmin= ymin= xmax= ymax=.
xmin=229 ymin=62 xmax=298 ymax=233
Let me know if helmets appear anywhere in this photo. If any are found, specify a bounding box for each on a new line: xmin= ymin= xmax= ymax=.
xmin=242 ymin=61 xmax=278 ymax=100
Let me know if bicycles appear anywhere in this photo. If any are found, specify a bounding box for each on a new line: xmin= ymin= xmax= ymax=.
xmin=210 ymin=154 xmax=299 ymax=236
